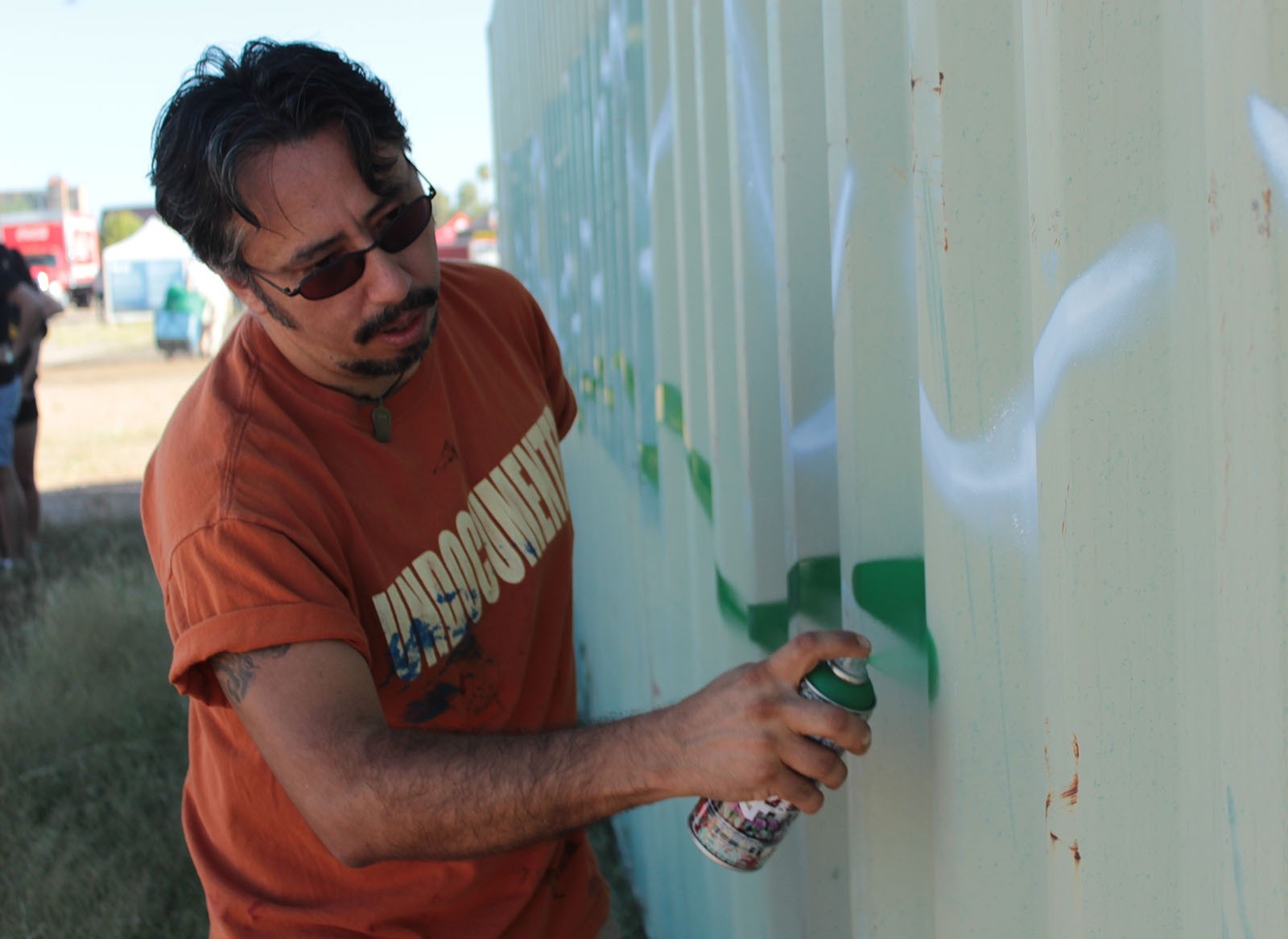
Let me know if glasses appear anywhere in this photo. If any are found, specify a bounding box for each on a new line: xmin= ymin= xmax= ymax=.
xmin=244 ymin=159 xmax=435 ymax=302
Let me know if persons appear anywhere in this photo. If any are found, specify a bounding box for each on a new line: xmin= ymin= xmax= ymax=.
xmin=135 ymin=33 xmax=879 ymax=938
xmin=0 ymin=244 xmax=66 ymax=580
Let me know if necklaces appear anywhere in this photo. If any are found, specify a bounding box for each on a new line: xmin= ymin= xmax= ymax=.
xmin=304 ymin=371 xmax=411 ymax=444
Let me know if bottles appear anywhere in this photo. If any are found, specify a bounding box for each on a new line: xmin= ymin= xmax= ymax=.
xmin=688 ymin=654 xmax=880 ymax=873
